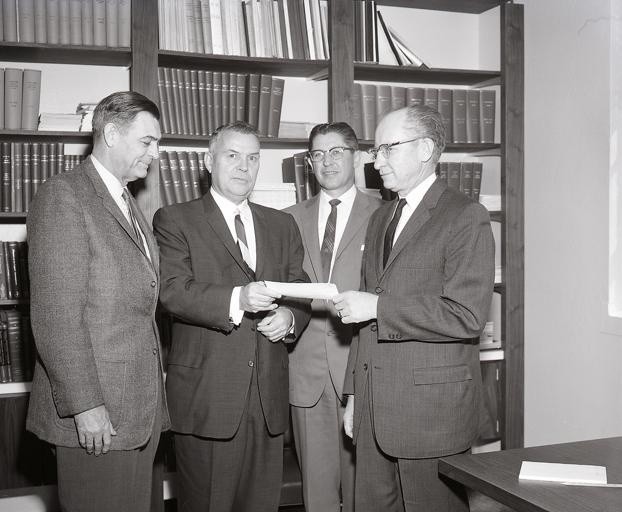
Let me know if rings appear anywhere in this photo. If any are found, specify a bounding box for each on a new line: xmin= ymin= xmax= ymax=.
xmin=338 ymin=309 xmax=343 ymax=319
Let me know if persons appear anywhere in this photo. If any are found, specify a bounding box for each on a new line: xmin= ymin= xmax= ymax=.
xmin=150 ymin=120 xmax=313 ymax=512
xmin=25 ymin=90 xmax=173 ymax=511
xmin=324 ymin=104 xmax=498 ymax=512
xmin=271 ymin=121 xmax=391 ymax=512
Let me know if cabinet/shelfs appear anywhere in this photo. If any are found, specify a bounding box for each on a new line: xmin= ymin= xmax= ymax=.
xmin=0 ymin=0 xmax=525 ymax=500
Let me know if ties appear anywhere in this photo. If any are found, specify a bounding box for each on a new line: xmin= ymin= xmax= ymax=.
xmin=122 ymin=188 xmax=158 ymax=272
xmin=234 ymin=214 xmax=251 ymax=267
xmin=319 ymin=199 xmax=341 ymax=283
xmin=381 ymin=198 xmax=407 ymax=268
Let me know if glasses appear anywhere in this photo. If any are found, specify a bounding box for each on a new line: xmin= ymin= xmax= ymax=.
xmin=367 ymin=138 xmax=420 ymax=159
xmin=310 ymin=146 xmax=354 ymax=162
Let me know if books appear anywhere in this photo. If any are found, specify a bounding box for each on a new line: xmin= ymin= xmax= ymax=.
xmin=477 ymin=320 xmax=496 ymax=348
xmin=518 ymin=459 xmax=609 ymax=486
xmin=0 ymin=238 xmax=38 ymax=384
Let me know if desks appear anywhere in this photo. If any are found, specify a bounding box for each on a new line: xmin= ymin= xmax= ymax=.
xmin=439 ymin=437 xmax=622 ymax=512
